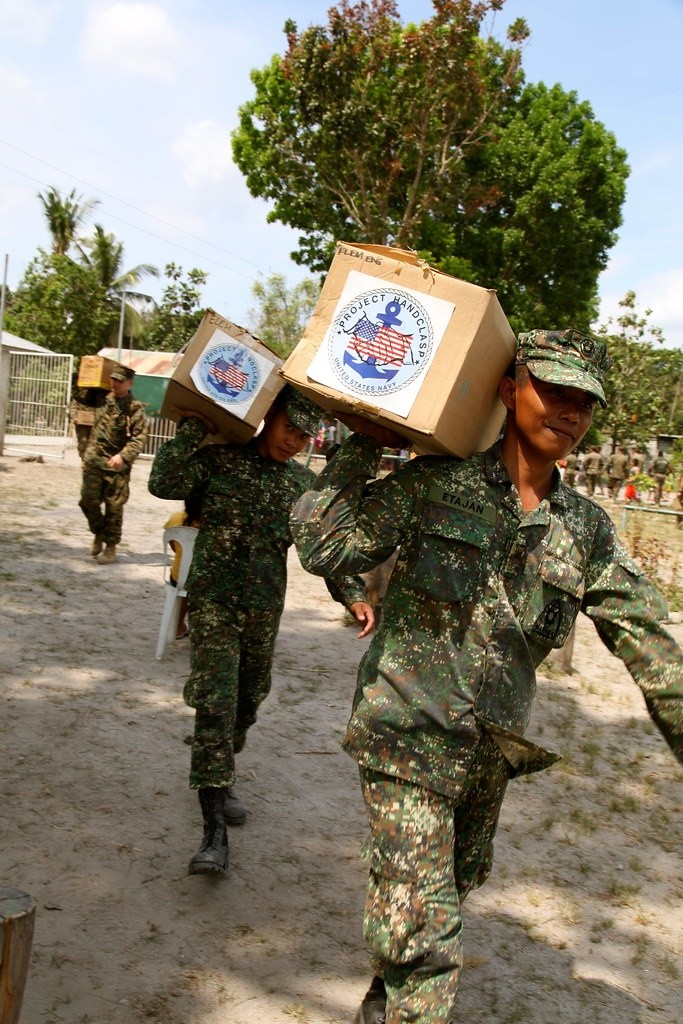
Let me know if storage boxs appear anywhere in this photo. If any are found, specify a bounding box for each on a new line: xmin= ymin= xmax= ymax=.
xmin=279 ymin=235 xmax=521 ymax=463
xmin=75 ymin=352 xmax=123 ymax=397
xmin=159 ymin=310 xmax=289 ymax=449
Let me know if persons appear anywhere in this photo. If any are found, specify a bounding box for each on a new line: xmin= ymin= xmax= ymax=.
xmin=629 ymin=447 xmax=645 ymax=490
xmin=581 ymin=443 xmax=603 ymax=501
xmin=290 ymin=330 xmax=683 ymax=1023
xmin=625 ymin=458 xmax=644 ymax=507
xmin=161 ymin=492 xmax=211 ymax=643
xmin=312 ymin=411 xmax=340 ymax=455
xmin=78 ymin=362 xmax=150 ymax=566
xmin=146 ymin=377 xmax=378 ymax=877
xmin=553 ymin=447 xmax=579 ymax=499
xmin=602 ymin=447 xmax=627 ymax=504
xmin=68 ymin=364 xmax=106 ymax=470
xmin=584 ymin=445 xmax=608 ymax=495
xmin=647 ymin=450 xmax=674 ymax=506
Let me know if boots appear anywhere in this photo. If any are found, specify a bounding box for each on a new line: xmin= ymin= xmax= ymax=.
xmin=187 ymin=788 xmax=230 ymax=874
xmin=98 ymin=545 xmax=117 ymax=563
xmin=220 ymin=787 xmax=247 ymax=825
xmin=90 ymin=534 xmax=103 ymax=554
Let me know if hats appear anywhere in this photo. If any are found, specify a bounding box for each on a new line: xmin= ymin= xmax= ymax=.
xmin=110 ymin=364 xmax=135 ymax=381
xmin=515 ymin=330 xmax=608 ymax=409
xmin=280 ymin=384 xmax=327 ymax=437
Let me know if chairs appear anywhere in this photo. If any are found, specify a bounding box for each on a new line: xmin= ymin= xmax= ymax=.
xmin=149 ymin=523 xmax=206 ymax=662
